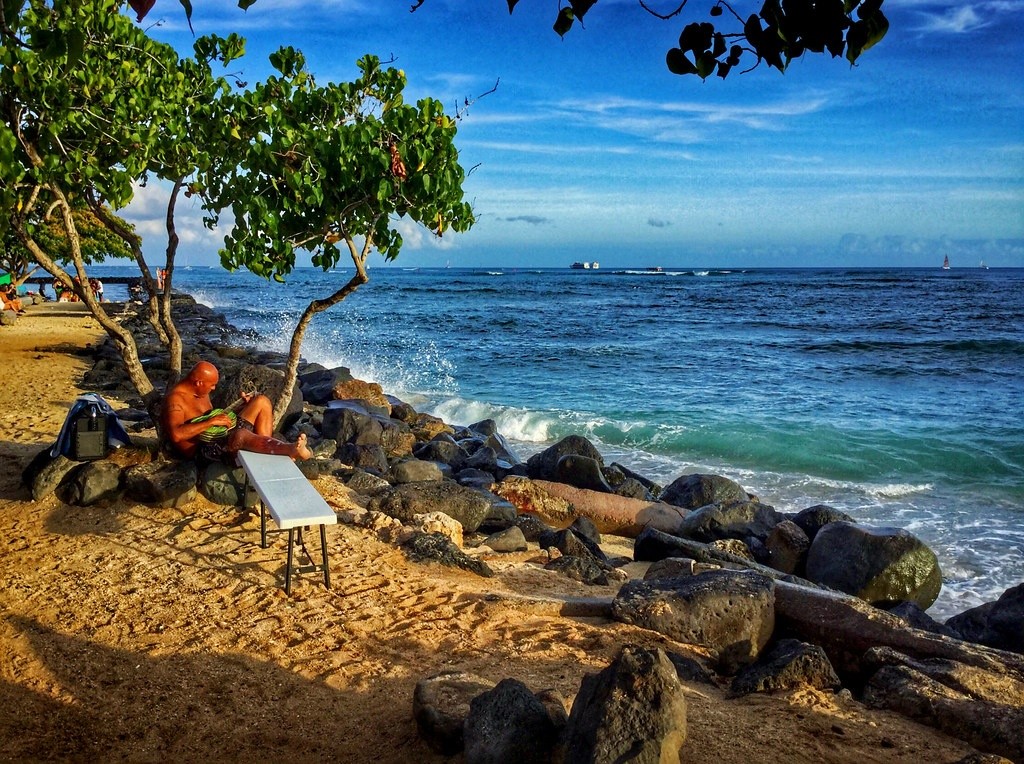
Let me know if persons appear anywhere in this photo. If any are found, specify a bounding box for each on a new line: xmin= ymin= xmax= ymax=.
xmin=0 ymin=274 xmax=104 ymax=318
xmin=166 ymin=360 xmax=310 ymax=463
xmin=155 ymin=267 xmax=166 ymax=290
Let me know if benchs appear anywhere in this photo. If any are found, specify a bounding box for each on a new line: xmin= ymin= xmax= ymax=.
xmin=0 ymin=297 xmax=33 ymax=325
xmin=238 ymin=450 xmax=339 ymax=596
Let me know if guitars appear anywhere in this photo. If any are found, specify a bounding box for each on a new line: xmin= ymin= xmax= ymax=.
xmin=190 ymin=386 xmax=257 ymax=444
